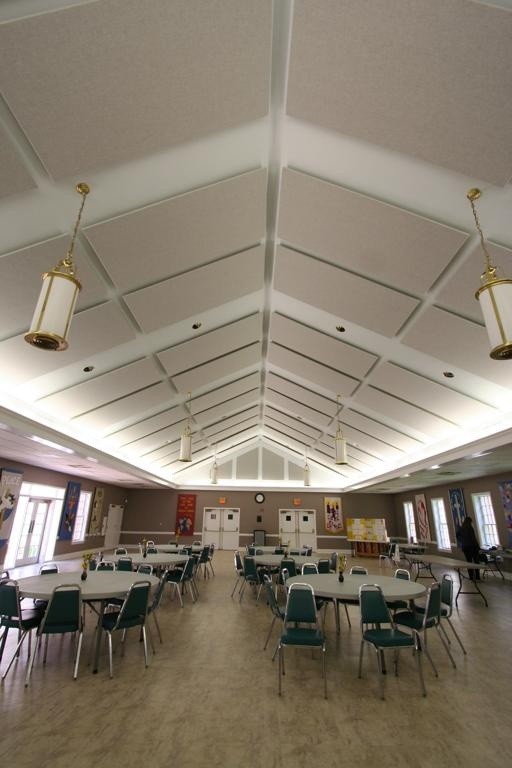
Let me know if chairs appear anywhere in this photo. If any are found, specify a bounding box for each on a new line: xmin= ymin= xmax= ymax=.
xmin=477 ymin=553 xmax=505 ymax=580
xmin=233 ymin=538 xmax=466 ymax=701
xmin=0 ymin=539 xmax=216 ymax=687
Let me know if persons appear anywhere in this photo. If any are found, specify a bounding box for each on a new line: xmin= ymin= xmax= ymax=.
xmin=180 ymin=516 xmax=192 ymax=532
xmin=455 ymin=516 xmax=484 ymax=581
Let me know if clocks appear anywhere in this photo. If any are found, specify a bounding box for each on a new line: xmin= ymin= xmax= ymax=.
xmin=255 ymin=493 xmax=265 ymax=503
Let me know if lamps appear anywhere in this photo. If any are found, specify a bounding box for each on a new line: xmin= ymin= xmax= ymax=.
xmin=303 ymin=443 xmax=311 ymax=486
xmin=466 ymin=189 xmax=512 ymax=361
xmin=212 ymin=443 xmax=218 ymax=483
xmin=25 ymin=182 xmax=89 ymax=353
xmin=180 ymin=391 xmax=194 ymax=462
xmin=335 ymin=396 xmax=348 ymax=465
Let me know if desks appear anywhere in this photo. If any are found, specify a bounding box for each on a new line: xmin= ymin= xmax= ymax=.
xmin=406 ymin=554 xmax=489 ymax=607
